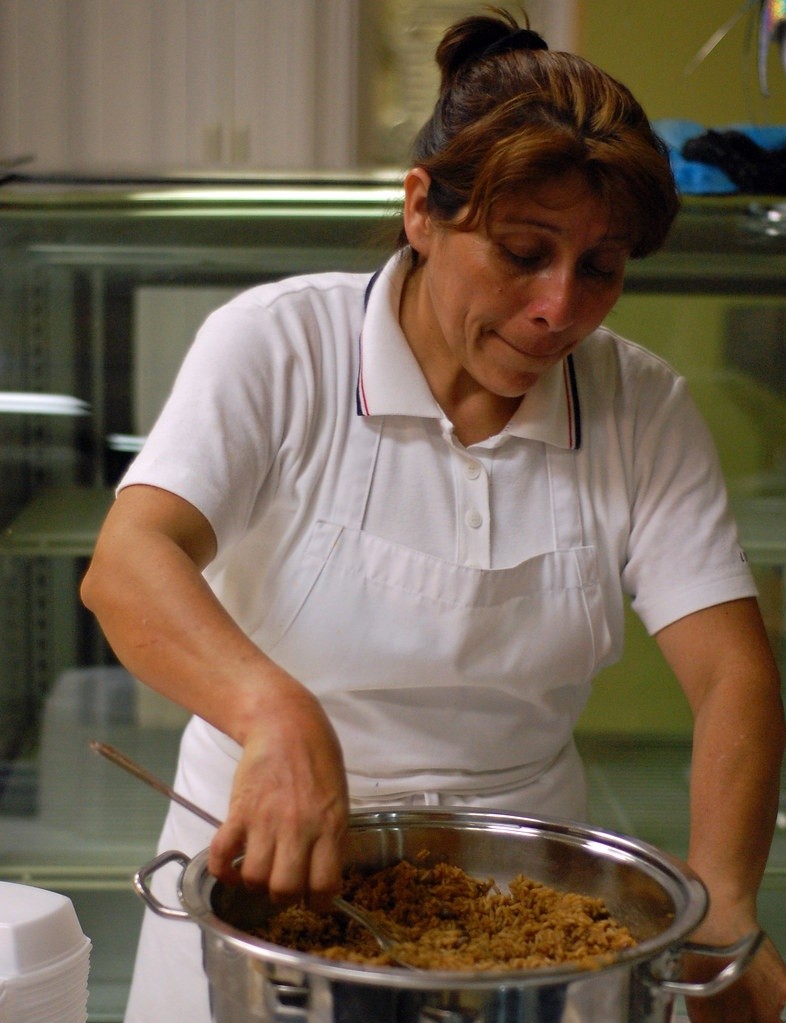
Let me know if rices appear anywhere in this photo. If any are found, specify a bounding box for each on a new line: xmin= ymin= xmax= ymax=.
xmin=249 ymin=862 xmax=639 ymax=975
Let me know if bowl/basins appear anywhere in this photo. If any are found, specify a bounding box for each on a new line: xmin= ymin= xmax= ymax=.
xmin=0 ymin=880 xmax=94 ymax=1023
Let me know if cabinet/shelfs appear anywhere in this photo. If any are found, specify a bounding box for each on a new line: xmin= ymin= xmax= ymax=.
xmin=0 ymin=151 xmax=786 ymax=818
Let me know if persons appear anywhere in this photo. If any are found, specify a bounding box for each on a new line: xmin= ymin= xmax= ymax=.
xmin=80 ymin=11 xmax=786 ymax=1023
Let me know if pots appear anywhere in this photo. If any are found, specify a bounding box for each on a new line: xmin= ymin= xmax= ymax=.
xmin=130 ymin=806 xmax=768 ymax=1023
